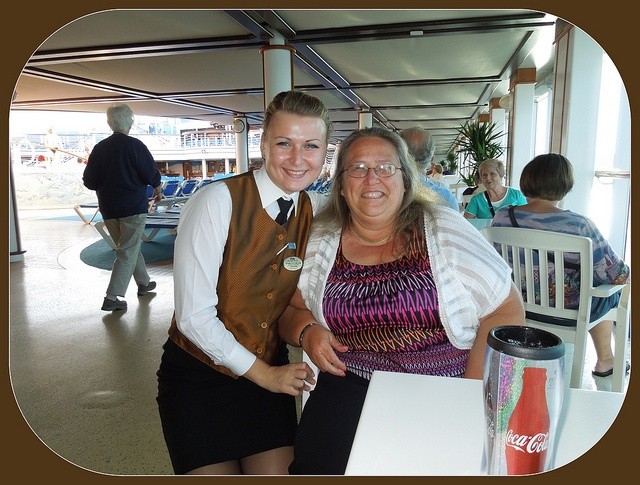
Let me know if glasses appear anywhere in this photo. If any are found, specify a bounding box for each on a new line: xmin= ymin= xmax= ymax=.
xmin=340 ymin=163 xmax=402 ymax=178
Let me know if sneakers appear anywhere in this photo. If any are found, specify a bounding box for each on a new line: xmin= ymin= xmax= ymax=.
xmin=138 ymin=282 xmax=156 ymax=295
xmin=101 ymin=297 xmax=127 ymax=311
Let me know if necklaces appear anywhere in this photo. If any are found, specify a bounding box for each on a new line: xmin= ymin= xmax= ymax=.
xmin=351 ymin=217 xmax=395 ymax=244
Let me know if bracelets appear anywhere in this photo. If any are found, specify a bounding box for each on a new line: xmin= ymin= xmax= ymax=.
xmin=298 ymin=322 xmax=321 ymax=348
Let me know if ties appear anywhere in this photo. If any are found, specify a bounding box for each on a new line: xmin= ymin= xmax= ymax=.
xmin=275 ymin=197 xmax=293 ymax=225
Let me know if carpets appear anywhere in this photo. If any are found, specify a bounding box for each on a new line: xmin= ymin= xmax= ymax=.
xmin=79 ymin=227 xmax=177 ymax=271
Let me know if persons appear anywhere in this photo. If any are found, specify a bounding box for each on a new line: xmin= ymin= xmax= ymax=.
xmin=278 ymin=128 xmax=527 ymax=475
xmin=426 ymin=159 xmax=454 ymax=179
xmin=82 ymin=104 xmax=162 ymax=313
xmin=399 ymin=128 xmax=459 ymax=212
xmin=462 ymin=159 xmax=527 ymax=219
xmin=491 ymin=154 xmax=630 ymax=378
xmin=155 ymin=92 xmax=330 ymax=477
xmin=463 ymin=171 xmax=482 ymax=209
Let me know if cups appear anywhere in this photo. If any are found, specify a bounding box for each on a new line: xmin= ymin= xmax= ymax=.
xmin=479 ymin=324 xmax=570 ymax=476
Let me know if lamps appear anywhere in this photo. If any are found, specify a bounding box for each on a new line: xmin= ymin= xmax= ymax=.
xmin=232 ymin=119 xmax=245 ymax=133
xmin=499 ymin=94 xmax=513 ymax=111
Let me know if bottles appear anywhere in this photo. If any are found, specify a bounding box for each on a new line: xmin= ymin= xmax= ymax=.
xmin=505 ymin=366 xmax=551 ymax=475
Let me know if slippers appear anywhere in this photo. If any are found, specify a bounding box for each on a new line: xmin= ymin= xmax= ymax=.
xmin=592 ymin=361 xmax=630 ymax=377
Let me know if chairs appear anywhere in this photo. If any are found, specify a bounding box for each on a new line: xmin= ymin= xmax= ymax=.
xmin=305 ymin=178 xmax=332 ymax=195
xmin=75 ymin=171 xmax=237 ymax=251
xmin=481 ymin=226 xmax=630 ymax=393
